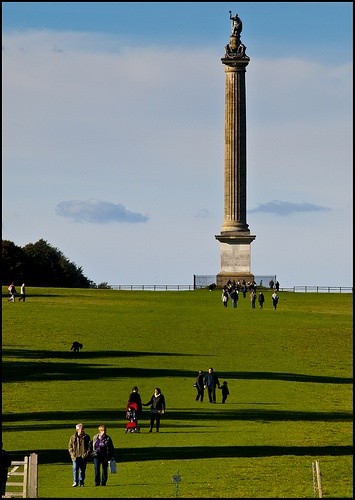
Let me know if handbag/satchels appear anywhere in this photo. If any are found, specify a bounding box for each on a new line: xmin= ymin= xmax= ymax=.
xmin=109 ymin=459 xmax=117 ymax=473
xmin=193 ymin=382 xmax=198 ymax=388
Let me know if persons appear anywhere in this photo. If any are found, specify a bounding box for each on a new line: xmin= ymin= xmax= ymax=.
xmin=275 ymin=281 xmax=279 ymax=291
xmin=204 ymin=367 xmax=220 ymax=403
xmin=208 ymin=283 xmax=217 ymax=292
xmin=9 ymin=282 xmax=16 ymax=302
xmin=219 ymin=382 xmax=230 ymax=403
xmin=128 ymin=386 xmax=143 ymax=430
xmin=67 ymin=423 xmax=91 ymax=487
xmin=143 ymin=388 xmax=165 ymax=433
xmin=2 ymin=442 xmax=11 ymax=495
xmin=195 ymin=371 xmax=204 ymax=402
xmin=229 ymin=11 xmax=242 ymax=37
xmin=272 ymin=291 xmax=279 ymax=310
xmin=269 ymin=279 xmax=274 ymax=289
xmin=222 ymin=279 xmax=257 ymax=308
xmin=71 ymin=342 xmax=83 ymax=352
xmin=260 ymin=280 xmax=263 ymax=286
xmin=126 ymin=407 xmax=136 ymax=420
xmin=90 ymin=424 xmax=114 ymax=486
xmin=258 ymin=292 xmax=264 ymax=309
xmin=19 ymin=283 xmax=26 ymax=302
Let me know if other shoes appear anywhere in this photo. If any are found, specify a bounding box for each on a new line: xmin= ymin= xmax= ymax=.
xmin=155 ymin=427 xmax=160 ymax=432
xmin=209 ymin=400 xmax=216 ymax=403
xmin=148 ymin=429 xmax=153 ymax=432
xmin=79 ymin=483 xmax=84 ymax=487
xmin=72 ymin=482 xmax=78 ymax=487
xmin=94 ymin=482 xmax=106 ymax=486
